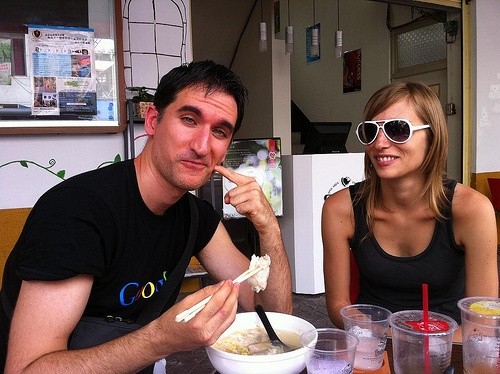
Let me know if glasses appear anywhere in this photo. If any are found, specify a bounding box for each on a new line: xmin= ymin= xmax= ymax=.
xmin=355 ymin=119 xmax=431 ymax=146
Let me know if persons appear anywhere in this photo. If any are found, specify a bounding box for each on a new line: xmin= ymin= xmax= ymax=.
xmin=0 ymin=58 xmax=293 ymax=374
xmin=321 ymin=80 xmax=499 ymax=343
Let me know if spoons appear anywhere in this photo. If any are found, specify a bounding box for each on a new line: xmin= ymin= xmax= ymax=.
xmin=254 ymin=304 xmax=295 ymax=353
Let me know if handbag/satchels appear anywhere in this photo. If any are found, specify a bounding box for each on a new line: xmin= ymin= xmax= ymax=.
xmin=69 ymin=316 xmax=152 ymax=374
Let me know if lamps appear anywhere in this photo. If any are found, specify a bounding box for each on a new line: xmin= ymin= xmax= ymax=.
xmin=311 ymin=0 xmax=320 ymax=55
xmin=259 ymin=0 xmax=268 ymax=52
xmin=335 ymin=0 xmax=343 ymax=56
xmin=285 ymin=0 xmax=295 ymax=56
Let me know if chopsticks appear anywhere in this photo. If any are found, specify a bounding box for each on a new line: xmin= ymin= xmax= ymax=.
xmin=174 ymin=265 xmax=263 ymax=323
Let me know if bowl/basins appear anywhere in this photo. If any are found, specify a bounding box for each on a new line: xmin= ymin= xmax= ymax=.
xmin=205 ymin=312 xmax=319 ymax=374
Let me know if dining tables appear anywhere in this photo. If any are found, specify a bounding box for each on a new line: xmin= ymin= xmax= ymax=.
xmin=213 ymin=331 xmax=500 ymax=374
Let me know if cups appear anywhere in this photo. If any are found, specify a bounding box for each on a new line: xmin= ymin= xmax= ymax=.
xmin=299 ymin=327 xmax=359 ymax=374
xmin=339 ymin=303 xmax=392 ymax=371
xmin=457 ymin=296 xmax=500 ymax=374
xmin=387 ymin=310 xmax=458 ymax=374
